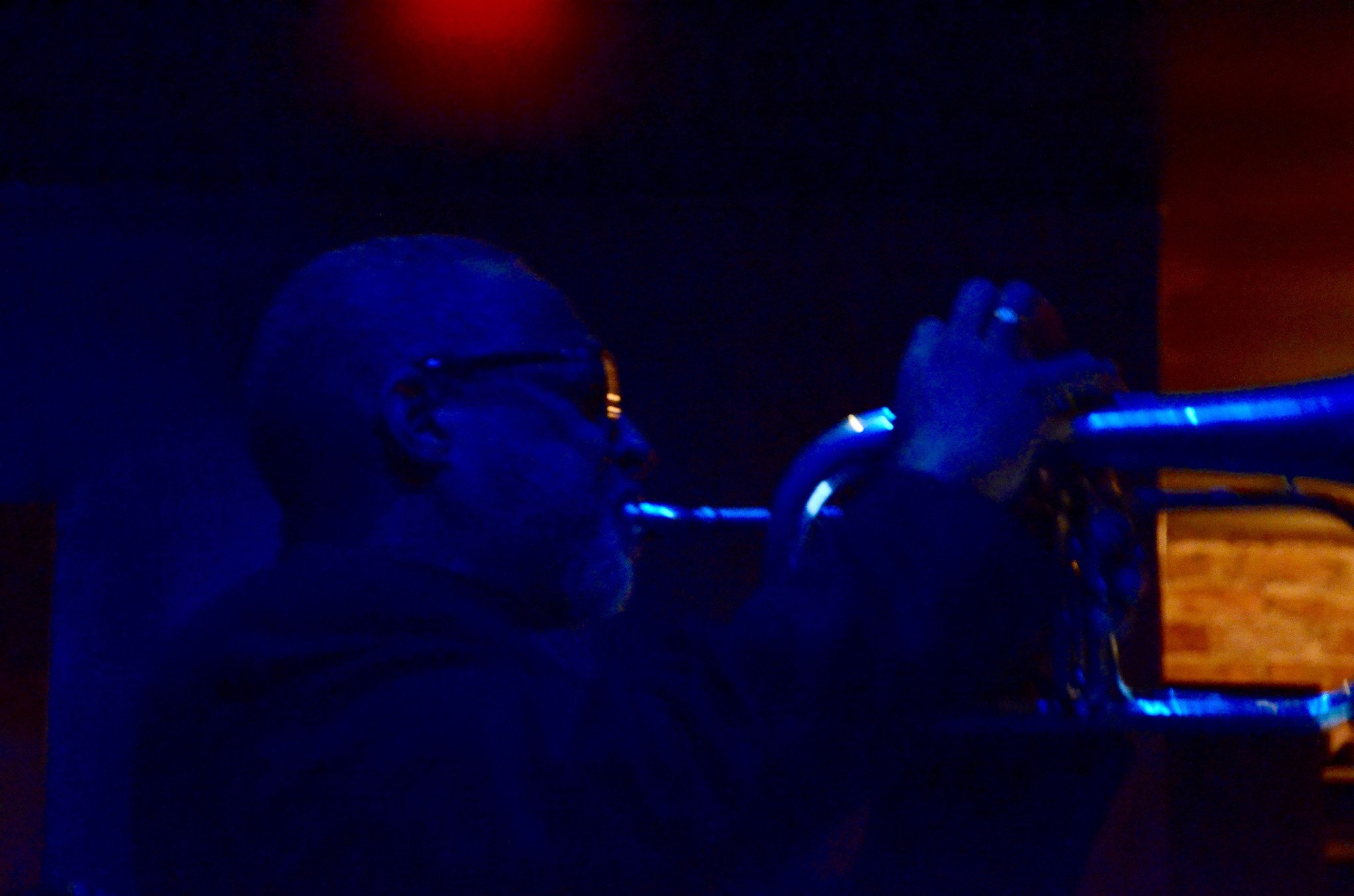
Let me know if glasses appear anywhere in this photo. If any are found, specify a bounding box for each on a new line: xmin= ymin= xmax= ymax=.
xmin=409 ymin=349 xmax=621 ymax=440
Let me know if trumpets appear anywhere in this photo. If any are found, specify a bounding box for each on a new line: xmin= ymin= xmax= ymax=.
xmin=617 ymin=376 xmax=1354 ymax=737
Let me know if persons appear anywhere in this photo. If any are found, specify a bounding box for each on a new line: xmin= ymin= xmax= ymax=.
xmin=132 ymin=236 xmax=1118 ymax=896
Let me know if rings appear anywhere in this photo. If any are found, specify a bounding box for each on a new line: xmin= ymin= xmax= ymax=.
xmin=995 ymin=307 xmax=1020 ymax=324
